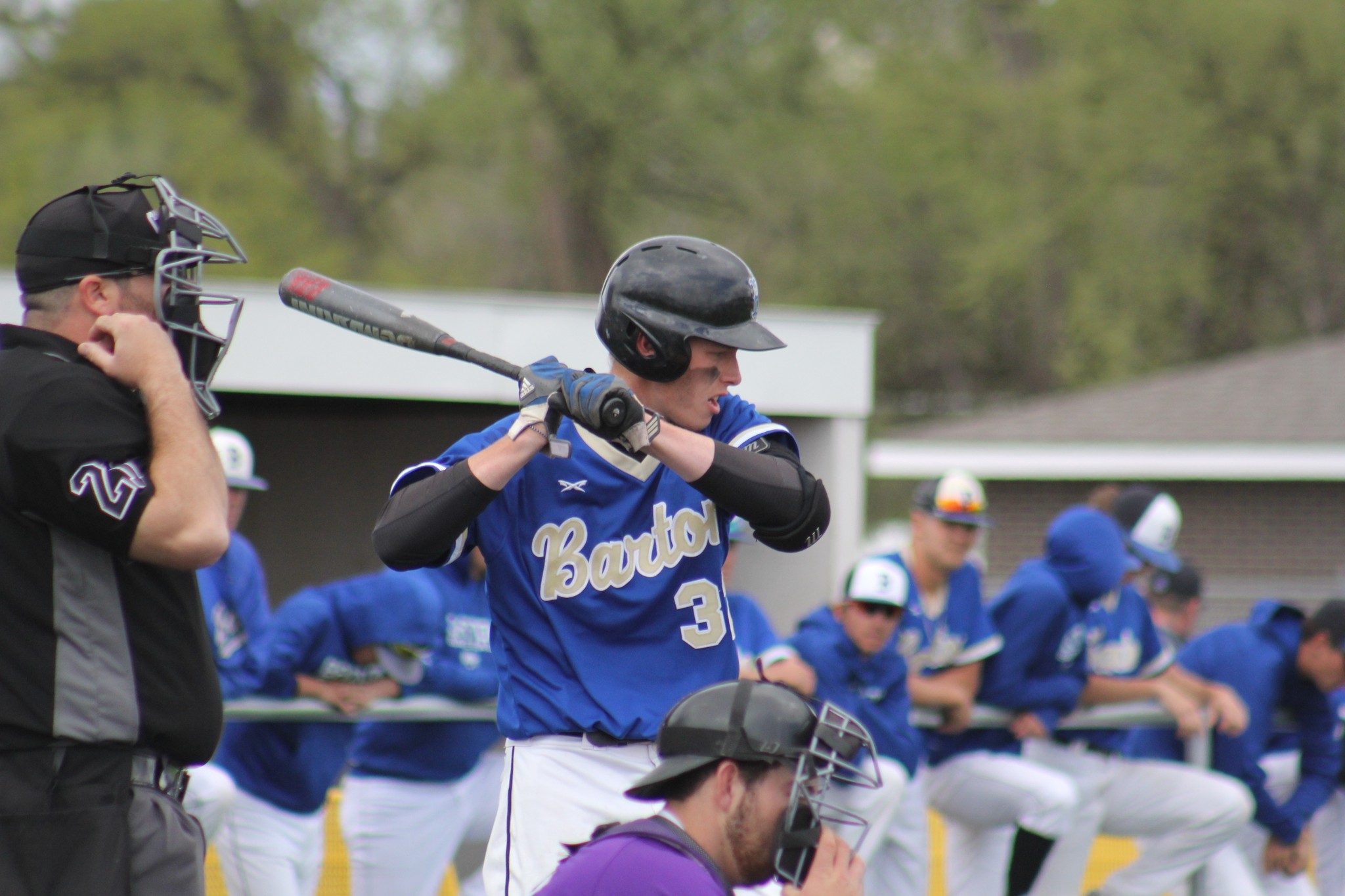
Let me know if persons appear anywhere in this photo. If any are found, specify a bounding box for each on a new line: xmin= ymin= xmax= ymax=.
xmin=0 ymin=173 xmax=249 ymax=896
xmin=209 ymin=564 xmax=448 ymax=896
xmin=341 ymin=545 xmax=508 ymax=896
xmin=373 ymin=236 xmax=830 ymax=896
xmin=534 ymin=677 xmax=883 ymax=896
xmin=719 ymin=471 xmax=1345 ymax=896
xmin=181 ymin=426 xmax=273 ymax=843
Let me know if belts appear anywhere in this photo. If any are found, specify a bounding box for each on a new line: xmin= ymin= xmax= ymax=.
xmin=130 ymin=755 xmax=190 ymax=805
xmin=556 ymin=730 xmax=654 ymax=748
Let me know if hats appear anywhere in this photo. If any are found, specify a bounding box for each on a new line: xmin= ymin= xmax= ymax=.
xmin=914 ymin=467 xmax=989 ymax=528
xmin=1112 ymin=488 xmax=1183 ymax=574
xmin=844 ymin=556 xmax=910 ymax=610
xmin=14 ymin=171 xmax=216 ymax=293
xmin=209 ymin=427 xmax=270 ymax=491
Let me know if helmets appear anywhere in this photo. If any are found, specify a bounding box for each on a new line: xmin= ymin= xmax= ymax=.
xmin=623 ymin=681 xmax=832 ymax=802
xmin=594 ymin=235 xmax=788 ymax=382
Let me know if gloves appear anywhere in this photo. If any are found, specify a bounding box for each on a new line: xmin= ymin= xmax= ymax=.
xmin=508 ymin=354 xmax=573 ymax=460
xmin=561 ymin=368 xmax=664 ymax=463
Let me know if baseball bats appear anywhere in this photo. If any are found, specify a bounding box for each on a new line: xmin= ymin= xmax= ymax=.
xmin=279 ymin=267 xmax=626 ymax=428
